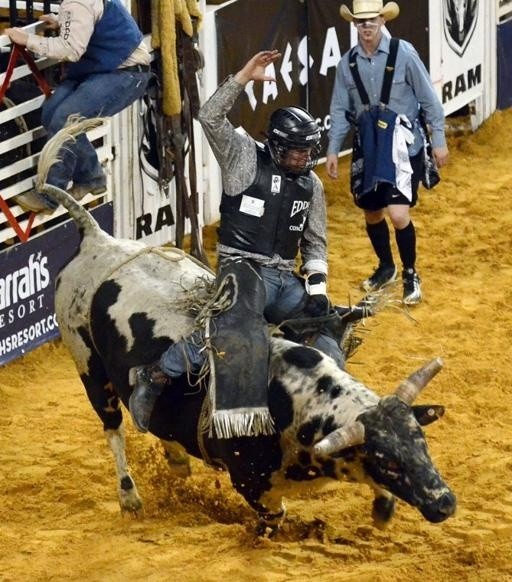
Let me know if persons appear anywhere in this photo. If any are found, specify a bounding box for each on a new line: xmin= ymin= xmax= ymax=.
xmin=325 ymin=0 xmax=448 ymax=306
xmin=3 ymin=0 xmax=153 ymax=217
xmin=128 ymin=48 xmax=346 ymax=433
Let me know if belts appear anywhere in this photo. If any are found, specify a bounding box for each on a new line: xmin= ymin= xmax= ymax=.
xmin=126 ymin=63 xmax=150 ymax=73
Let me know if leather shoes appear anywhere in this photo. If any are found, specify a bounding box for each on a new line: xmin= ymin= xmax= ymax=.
xmin=66 ymin=183 xmax=108 ymax=200
xmin=14 ymin=190 xmax=56 ymax=215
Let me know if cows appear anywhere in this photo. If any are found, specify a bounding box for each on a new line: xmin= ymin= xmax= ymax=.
xmin=35 ymin=113 xmax=457 ymax=539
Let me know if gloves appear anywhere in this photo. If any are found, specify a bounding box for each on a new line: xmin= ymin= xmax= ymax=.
xmin=305 ymin=273 xmax=328 ymax=316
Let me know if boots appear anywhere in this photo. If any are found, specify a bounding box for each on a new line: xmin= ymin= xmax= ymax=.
xmin=129 ymin=363 xmax=170 ymax=433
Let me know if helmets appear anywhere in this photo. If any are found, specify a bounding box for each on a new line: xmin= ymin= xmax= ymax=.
xmin=266 ymin=106 xmax=323 ymax=177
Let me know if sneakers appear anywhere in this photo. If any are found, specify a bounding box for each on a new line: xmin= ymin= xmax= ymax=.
xmin=401 ymin=269 xmax=422 ymax=305
xmin=362 ymin=262 xmax=399 ymax=292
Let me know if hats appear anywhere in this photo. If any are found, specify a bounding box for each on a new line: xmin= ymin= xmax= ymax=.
xmin=340 ymin=0 xmax=399 ymax=21
